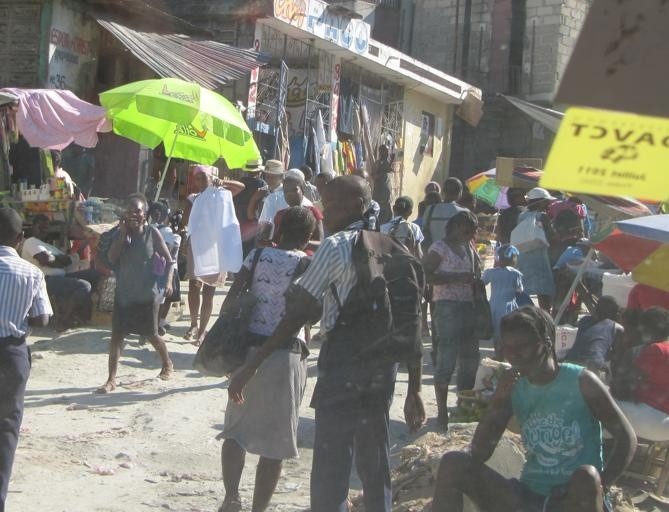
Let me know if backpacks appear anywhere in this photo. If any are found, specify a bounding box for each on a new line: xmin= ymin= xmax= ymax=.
xmin=327 ymin=226 xmax=426 ymax=370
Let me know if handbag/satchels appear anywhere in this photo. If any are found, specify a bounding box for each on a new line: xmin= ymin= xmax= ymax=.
xmin=468 ymin=301 xmax=493 ymax=340
xmin=194 ymin=292 xmax=258 ymax=378
xmin=510 ymin=218 xmax=548 ymax=253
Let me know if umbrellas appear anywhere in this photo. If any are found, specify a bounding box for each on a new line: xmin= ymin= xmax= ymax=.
xmin=98 ymin=77 xmax=261 ymax=201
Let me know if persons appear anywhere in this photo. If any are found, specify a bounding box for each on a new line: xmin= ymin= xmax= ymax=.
xmin=0 ymin=207 xmax=53 ymax=511
xmin=432 ymin=307 xmax=637 ymax=512
xmin=227 ymin=175 xmax=425 ymax=512
xmin=22 ymin=145 xmax=669 ymax=422
xmin=219 ymin=208 xmax=323 ymax=512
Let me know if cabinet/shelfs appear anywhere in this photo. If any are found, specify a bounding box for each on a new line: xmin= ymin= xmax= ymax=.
xmin=11 ymin=199 xmax=73 ymax=252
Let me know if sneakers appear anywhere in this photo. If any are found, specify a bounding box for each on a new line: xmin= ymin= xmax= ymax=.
xmin=160 ymin=364 xmax=173 ymax=381
xmin=185 ymin=324 xmax=207 ymax=346
xmin=98 ymin=380 xmax=116 ymax=393
xmin=55 ymin=319 xmax=69 ymax=331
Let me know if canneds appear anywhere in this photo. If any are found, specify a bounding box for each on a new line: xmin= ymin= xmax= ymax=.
xmin=55 ymin=177 xmax=70 ymax=212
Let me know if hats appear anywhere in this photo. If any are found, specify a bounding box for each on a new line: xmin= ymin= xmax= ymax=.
xmin=525 ymin=189 xmax=558 ymax=201
xmin=194 ymin=165 xmax=210 ymax=175
xmin=263 ymin=160 xmax=284 ymax=175
xmin=243 ymin=159 xmax=261 ymax=172
